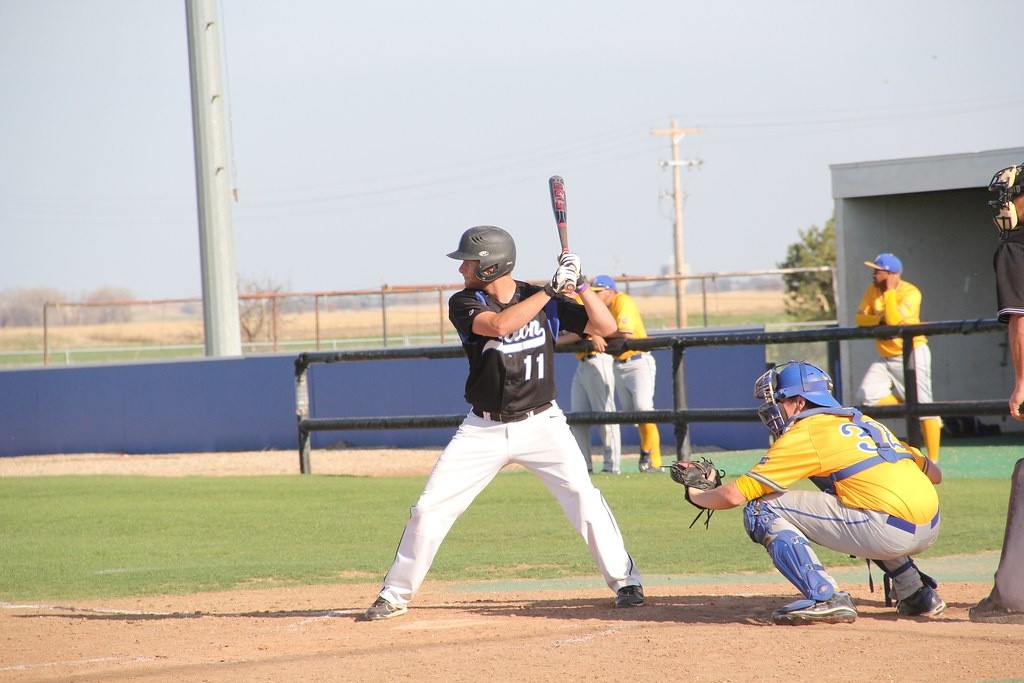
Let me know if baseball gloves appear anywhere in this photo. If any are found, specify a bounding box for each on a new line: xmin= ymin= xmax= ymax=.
xmin=670 ymin=460 xmax=724 ymax=509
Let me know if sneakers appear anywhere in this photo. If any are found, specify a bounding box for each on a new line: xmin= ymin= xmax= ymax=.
xmin=969 ymin=598 xmax=1023 ymax=625
xmin=771 ymin=590 xmax=857 ymax=626
xmin=637 ymin=447 xmax=650 ymax=471
xmin=896 ymin=584 xmax=946 ymax=617
xmin=611 ymin=584 xmax=644 ymax=606
xmin=363 ymin=597 xmax=408 ymax=620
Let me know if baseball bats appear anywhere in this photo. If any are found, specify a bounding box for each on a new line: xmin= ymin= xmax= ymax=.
xmin=549 ymin=175 xmax=576 ymax=292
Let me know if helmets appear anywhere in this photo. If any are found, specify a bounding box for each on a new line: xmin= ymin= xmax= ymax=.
xmin=995 ymin=165 xmax=1024 ymax=205
xmin=774 ymin=365 xmax=842 ymax=409
xmin=446 ymin=226 xmax=517 ymax=282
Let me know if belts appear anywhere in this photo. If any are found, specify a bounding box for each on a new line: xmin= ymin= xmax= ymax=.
xmin=886 ymin=507 xmax=940 ymax=535
xmin=616 ymin=354 xmax=641 ymax=364
xmin=580 ymin=354 xmax=596 ymax=362
xmin=470 ymin=402 xmax=554 ymax=424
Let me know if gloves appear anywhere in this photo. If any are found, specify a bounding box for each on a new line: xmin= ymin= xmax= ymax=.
xmin=545 ymin=266 xmax=576 ymax=297
xmin=554 ymin=253 xmax=583 ymax=286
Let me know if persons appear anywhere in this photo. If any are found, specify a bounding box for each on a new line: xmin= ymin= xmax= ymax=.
xmin=669 ymin=360 xmax=948 ymax=623
xmin=364 ymin=225 xmax=643 ymax=618
xmin=969 ymin=164 xmax=1024 ymax=624
xmin=559 ymin=275 xmax=664 ymax=473
xmin=856 ymin=254 xmax=941 ymax=466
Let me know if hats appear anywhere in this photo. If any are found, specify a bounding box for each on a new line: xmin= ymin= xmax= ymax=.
xmin=589 ymin=275 xmax=614 ymax=292
xmin=863 ymin=253 xmax=904 ymax=273
